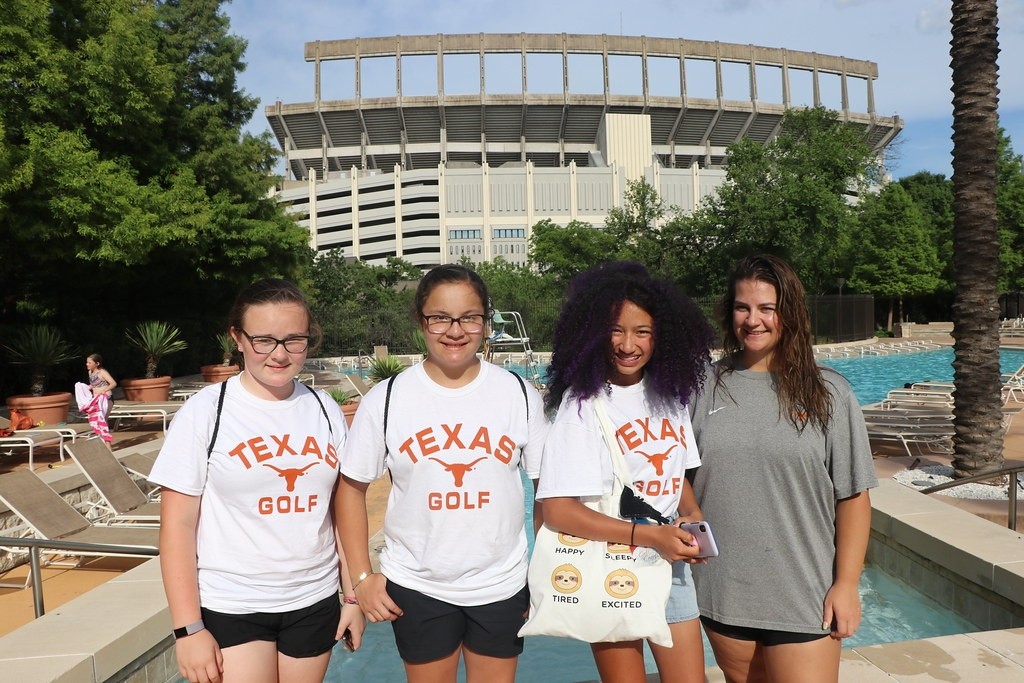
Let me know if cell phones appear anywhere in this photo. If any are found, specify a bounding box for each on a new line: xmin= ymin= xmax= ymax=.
xmin=679 ymin=521 xmax=719 ymax=558
xmin=342 ymin=628 xmax=354 ymax=653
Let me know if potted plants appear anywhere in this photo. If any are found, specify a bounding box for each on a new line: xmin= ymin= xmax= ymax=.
xmin=329 ymin=386 xmax=362 ymax=429
xmin=119 ymin=322 xmax=187 ymax=423
xmin=6 ymin=322 xmax=84 ymax=447
xmin=200 ymin=331 xmax=243 ymax=384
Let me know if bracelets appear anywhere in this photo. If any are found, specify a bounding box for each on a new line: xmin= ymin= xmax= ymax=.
xmin=631 ymin=523 xmax=636 ymax=546
xmin=173 ymin=619 xmax=204 ymax=640
xmin=352 ymin=570 xmax=373 ymax=590
xmin=343 ymin=596 xmax=359 ymax=605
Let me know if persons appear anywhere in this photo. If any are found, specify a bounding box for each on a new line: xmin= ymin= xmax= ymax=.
xmin=333 ymin=264 xmax=552 ymax=683
xmin=534 ymin=261 xmax=717 ymax=683
xmin=674 ymin=254 xmax=879 ymax=683
xmin=86 ymin=354 xmax=117 ymax=448
xmin=145 ymin=278 xmax=367 ymax=683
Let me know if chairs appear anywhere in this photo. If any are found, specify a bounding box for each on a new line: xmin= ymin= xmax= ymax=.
xmin=292 ymin=353 xmax=424 ymax=389
xmin=491 ymin=308 xmax=514 ymax=339
xmin=1000 ymin=317 xmax=1024 ymax=328
xmin=808 ymin=339 xmax=943 ymax=361
xmin=0 ymin=380 xmax=216 ymax=591
xmin=861 ymin=360 xmax=1024 ymax=457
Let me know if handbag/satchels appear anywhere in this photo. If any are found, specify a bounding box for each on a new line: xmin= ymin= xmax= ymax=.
xmin=517 ymin=396 xmax=674 ymax=649
xmin=9 ymin=411 xmax=33 ymax=430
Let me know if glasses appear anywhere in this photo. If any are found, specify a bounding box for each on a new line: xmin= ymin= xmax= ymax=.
xmin=421 ymin=312 xmax=486 ymax=334
xmin=241 ymin=328 xmax=312 ymax=354
